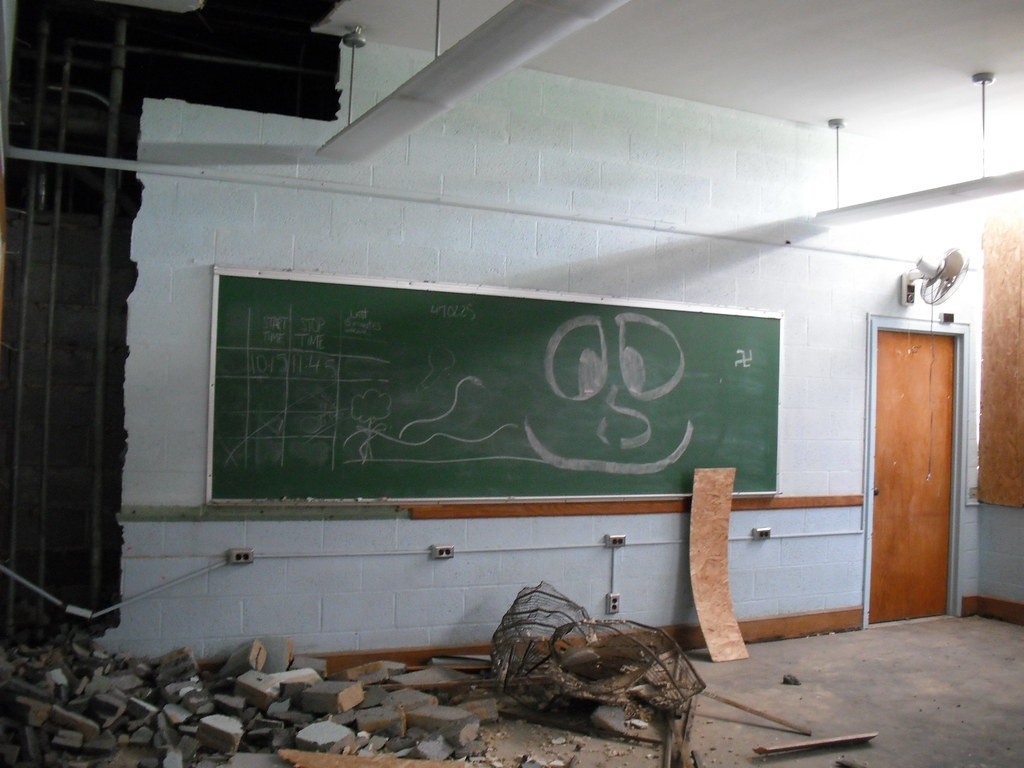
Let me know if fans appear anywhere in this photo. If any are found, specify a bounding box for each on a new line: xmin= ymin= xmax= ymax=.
xmin=900 ymin=248 xmax=969 ymax=306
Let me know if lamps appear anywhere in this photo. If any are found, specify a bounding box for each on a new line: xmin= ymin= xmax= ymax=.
xmin=814 ymin=71 xmax=1024 ymax=226
xmin=316 ymin=0 xmax=628 ymax=163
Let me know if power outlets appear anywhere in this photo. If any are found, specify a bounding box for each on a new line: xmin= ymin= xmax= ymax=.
xmin=230 ymin=548 xmax=254 ymax=564
xmin=606 ymin=593 xmax=619 ymax=614
xmin=606 ymin=534 xmax=626 ymax=547
xmin=752 ymin=527 xmax=771 ymax=540
xmin=431 ymin=544 xmax=454 ymax=559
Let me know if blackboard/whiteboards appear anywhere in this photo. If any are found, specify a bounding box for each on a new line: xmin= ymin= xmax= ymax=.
xmin=205 ymin=264 xmax=787 ymax=508
